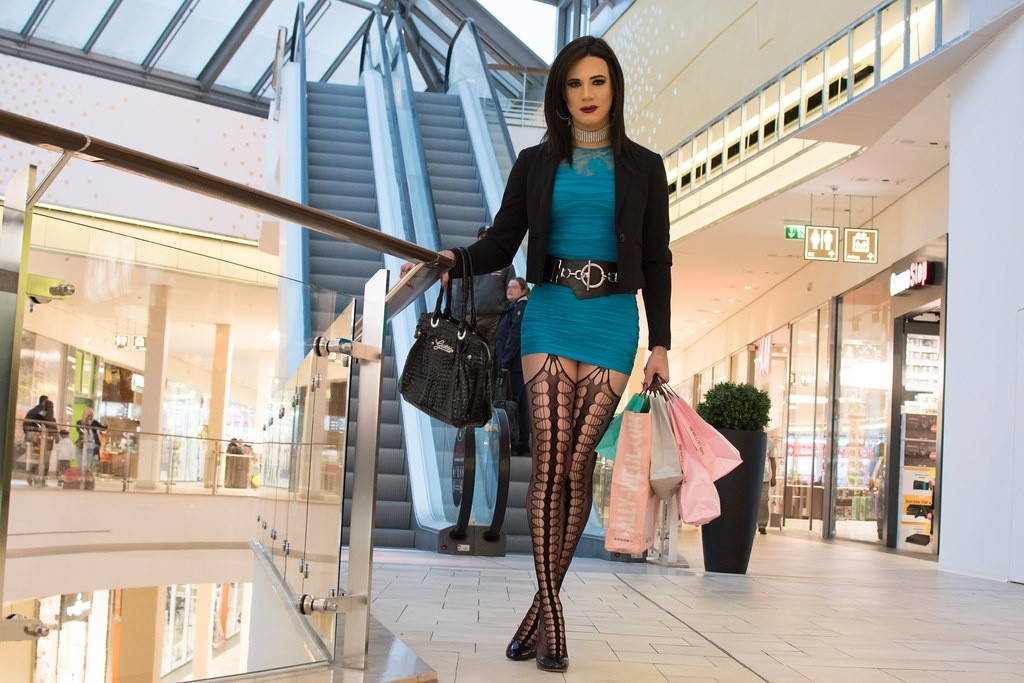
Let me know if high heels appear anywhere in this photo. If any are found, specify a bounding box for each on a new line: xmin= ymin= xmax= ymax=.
xmin=536 ymin=619 xmax=570 ymax=673
xmin=506 ymin=640 xmax=537 ymax=661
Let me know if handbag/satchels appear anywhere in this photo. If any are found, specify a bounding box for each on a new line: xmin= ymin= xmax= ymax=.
xmin=593 ymin=373 xmax=743 ymax=554
xmin=398 ymin=246 xmax=493 ymax=429
xmin=494 ymin=368 xmax=520 ymax=440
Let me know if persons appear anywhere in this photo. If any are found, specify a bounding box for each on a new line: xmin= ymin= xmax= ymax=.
xmin=453 ymin=224 xmax=517 ymax=359
xmin=227 ymin=438 xmax=241 ymax=453
xmin=399 ymin=35 xmax=674 ymax=673
xmin=76 ymin=407 xmax=109 ymax=480
xmin=755 ymin=435 xmax=776 ymax=535
xmin=493 ymin=277 xmax=532 ymax=458
xmin=22 ymin=396 xmax=59 ymax=486
xmin=55 ymin=430 xmax=75 ymax=486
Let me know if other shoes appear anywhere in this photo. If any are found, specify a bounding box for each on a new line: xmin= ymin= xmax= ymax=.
xmin=26 ymin=470 xmax=33 ymax=487
xmin=512 ymin=444 xmax=531 ymax=456
xmin=58 ymin=480 xmax=63 ymax=486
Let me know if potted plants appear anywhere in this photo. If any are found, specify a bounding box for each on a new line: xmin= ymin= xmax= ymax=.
xmin=694 ymin=380 xmax=775 ymax=576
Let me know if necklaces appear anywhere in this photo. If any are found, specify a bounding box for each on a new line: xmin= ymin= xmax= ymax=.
xmin=570 ymin=123 xmax=611 ymax=144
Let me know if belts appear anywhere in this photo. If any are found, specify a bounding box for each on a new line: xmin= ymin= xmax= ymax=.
xmin=544 ymin=256 xmax=637 ymax=300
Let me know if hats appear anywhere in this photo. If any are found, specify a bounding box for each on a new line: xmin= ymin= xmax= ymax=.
xmin=478 ymin=225 xmax=491 ymax=235
